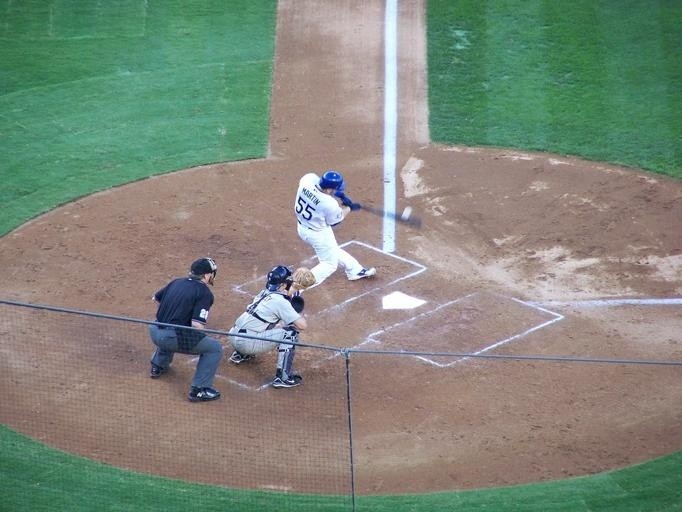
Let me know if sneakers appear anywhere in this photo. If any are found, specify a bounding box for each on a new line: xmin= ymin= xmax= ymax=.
xmin=150 ymin=363 xmax=169 ymax=378
xmin=188 ymin=386 xmax=221 ymax=401
xmin=348 ymin=265 xmax=378 ymax=281
xmin=272 ymin=374 xmax=303 ymax=389
xmin=229 ymin=349 xmax=256 ymax=365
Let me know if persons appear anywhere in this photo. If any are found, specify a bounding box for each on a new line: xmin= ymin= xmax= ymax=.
xmin=148 ymin=258 xmax=224 ymax=401
xmin=294 ymin=171 xmax=376 ymax=292
xmin=227 ymin=266 xmax=306 ymax=388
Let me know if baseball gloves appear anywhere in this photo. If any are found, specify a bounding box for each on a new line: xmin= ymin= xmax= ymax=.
xmin=290 ymin=265 xmax=315 ymax=290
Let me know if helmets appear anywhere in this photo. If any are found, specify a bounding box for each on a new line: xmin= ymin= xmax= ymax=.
xmin=318 ymin=169 xmax=346 ymax=192
xmin=266 ymin=265 xmax=294 ymax=291
xmin=191 ymin=256 xmax=217 ymax=274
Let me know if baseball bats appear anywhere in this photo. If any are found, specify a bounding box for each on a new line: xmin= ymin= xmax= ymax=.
xmin=341 ymin=201 xmax=421 ymax=232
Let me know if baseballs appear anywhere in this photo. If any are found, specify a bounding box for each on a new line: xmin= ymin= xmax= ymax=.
xmin=400 ymin=214 xmax=410 ymax=222
